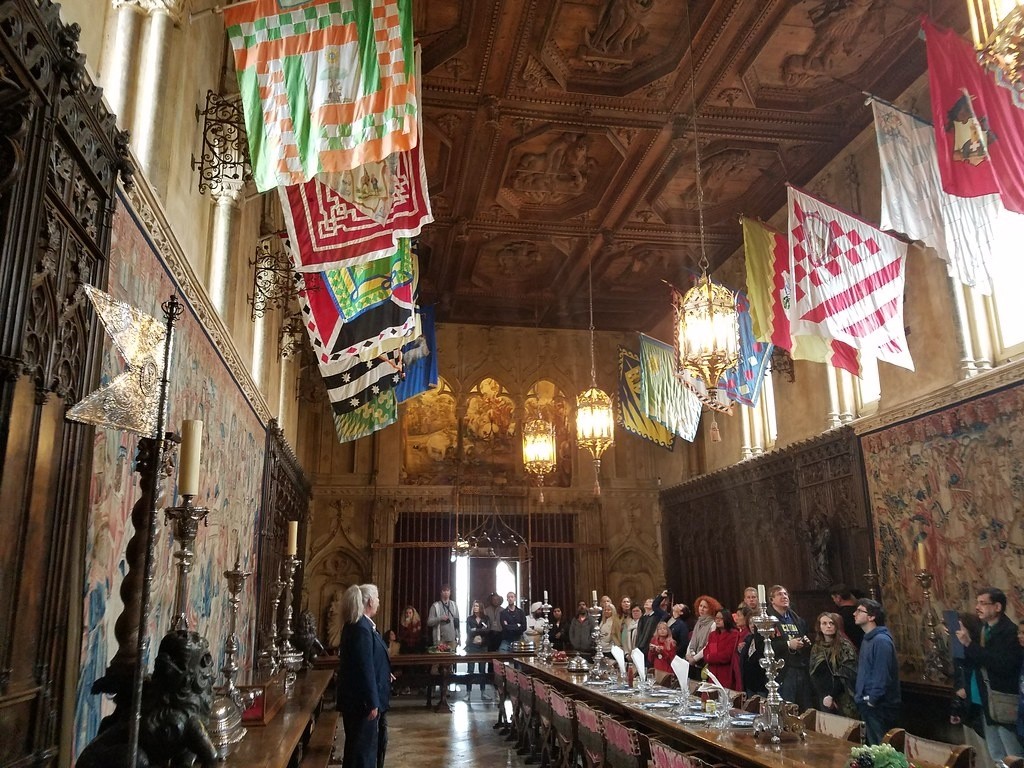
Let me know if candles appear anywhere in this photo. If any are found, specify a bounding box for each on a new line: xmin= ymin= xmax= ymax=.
xmin=917 ymin=542 xmax=928 ymax=570
xmin=757 ymin=584 xmax=766 ymax=603
xmin=178 ymin=419 xmax=204 ymax=495
xmin=287 ymin=520 xmax=299 ymax=556
xmin=592 ymin=590 xmax=598 ymax=600
xmin=544 ymin=590 xmax=548 ymax=599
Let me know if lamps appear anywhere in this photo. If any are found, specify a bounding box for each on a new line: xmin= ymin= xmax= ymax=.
xmin=450 ymin=418 xmax=534 ymax=559
xmin=669 ymin=0 xmax=744 ymax=443
xmin=520 ymin=246 xmax=558 ymax=506
xmin=574 ymin=105 xmax=615 ymax=495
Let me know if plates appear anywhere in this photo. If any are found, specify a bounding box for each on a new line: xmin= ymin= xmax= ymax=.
xmin=550 ymin=656 xmax=764 ymax=728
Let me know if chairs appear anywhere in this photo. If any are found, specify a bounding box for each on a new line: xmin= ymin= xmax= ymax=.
xmin=880 ymin=727 xmax=978 ymax=768
xmin=798 ymin=707 xmax=866 ymax=745
xmin=491 ymin=658 xmax=747 ymax=768
xmin=741 ymin=695 xmax=799 ymax=716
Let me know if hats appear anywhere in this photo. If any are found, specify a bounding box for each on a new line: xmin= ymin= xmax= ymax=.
xmin=829 ymin=584 xmax=855 ymax=601
xmin=531 ymin=602 xmax=542 ymax=612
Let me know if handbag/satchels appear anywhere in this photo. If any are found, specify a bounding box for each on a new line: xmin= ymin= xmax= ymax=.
xmin=987 ymin=689 xmax=1020 ymax=724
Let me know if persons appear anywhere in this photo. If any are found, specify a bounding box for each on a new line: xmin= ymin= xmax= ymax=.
xmin=802 ymin=510 xmax=835 ymax=590
xmin=854 ymin=598 xmax=902 ymax=748
xmin=569 ymin=585 xmax=866 ymax=748
xmin=335 ymin=583 xmax=396 ymax=768
xmin=398 ymin=605 xmax=429 ymax=696
xmin=548 ymin=608 xmax=571 ymax=652
xmin=703 ymin=608 xmax=739 ymax=689
xmin=328 ymin=592 xmax=344 ymax=655
xmin=382 ymin=629 xmax=403 ymax=698
xmin=484 ymin=593 xmax=527 ymax=701
xmin=647 ymin=622 xmax=678 ymax=673
xmin=463 ymin=600 xmax=493 ymax=702
xmin=522 ymin=601 xmax=549 ymax=644
xmin=808 ymin=612 xmax=862 ymax=720
xmin=950 ymin=587 xmax=1024 ymax=768
xmin=427 ymin=583 xmax=462 ymax=698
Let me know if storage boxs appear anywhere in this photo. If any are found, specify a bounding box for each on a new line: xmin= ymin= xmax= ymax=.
xmin=231 ymin=668 xmax=287 ymax=727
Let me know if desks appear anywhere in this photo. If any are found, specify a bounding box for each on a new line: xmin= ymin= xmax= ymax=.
xmin=512 ymin=655 xmax=945 ymax=768
xmin=899 ymin=669 xmax=954 ymax=708
xmin=311 ymin=649 xmax=592 ymax=714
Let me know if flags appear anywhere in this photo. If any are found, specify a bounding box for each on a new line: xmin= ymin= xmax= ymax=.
xmin=222 ymin=0 xmax=438 ymax=442
xmin=615 ymin=17 xmax=1024 ymax=452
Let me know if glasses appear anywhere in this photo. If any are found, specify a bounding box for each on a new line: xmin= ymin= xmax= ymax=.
xmin=714 ymin=617 xmax=724 ymax=620
xmin=855 ymin=609 xmax=870 ymax=615
xmin=977 ymin=602 xmax=994 ymax=608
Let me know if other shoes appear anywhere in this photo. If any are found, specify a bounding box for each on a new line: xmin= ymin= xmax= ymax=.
xmin=481 ymin=693 xmax=491 ymax=700
xmin=401 ymin=688 xmax=410 ymax=695
xmin=463 ymin=696 xmax=471 ymax=701
xmin=419 ymin=688 xmax=425 ymax=695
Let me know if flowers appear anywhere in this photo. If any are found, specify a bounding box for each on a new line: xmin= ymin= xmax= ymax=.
xmin=428 ymin=643 xmax=451 ymax=653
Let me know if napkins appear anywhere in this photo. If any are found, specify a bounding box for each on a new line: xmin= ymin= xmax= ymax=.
xmin=697 ymin=666 xmax=727 ymax=698
xmin=630 ymin=647 xmax=645 ymax=676
xmin=611 ymin=644 xmax=626 ymax=673
xmin=670 ymin=655 xmax=690 ymax=690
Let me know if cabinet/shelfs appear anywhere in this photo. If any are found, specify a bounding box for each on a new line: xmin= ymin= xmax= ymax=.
xmin=194 ymin=669 xmax=343 ymax=768
